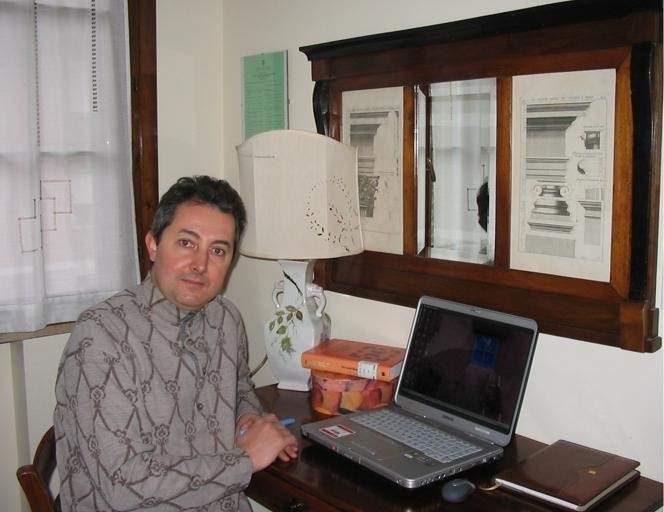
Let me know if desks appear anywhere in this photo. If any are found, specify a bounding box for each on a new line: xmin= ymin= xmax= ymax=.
xmin=237 ymin=385 xmax=664 ymax=510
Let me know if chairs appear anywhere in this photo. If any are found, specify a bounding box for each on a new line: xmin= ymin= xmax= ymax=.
xmin=14 ymin=426 xmax=59 ymax=511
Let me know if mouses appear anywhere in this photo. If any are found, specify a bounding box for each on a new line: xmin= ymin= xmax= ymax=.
xmin=442 ymin=479 xmax=477 ymax=503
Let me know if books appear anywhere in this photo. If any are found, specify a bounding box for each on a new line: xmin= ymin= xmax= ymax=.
xmin=490 ymin=436 xmax=642 ymax=511
xmin=300 ymin=338 xmax=407 ymax=383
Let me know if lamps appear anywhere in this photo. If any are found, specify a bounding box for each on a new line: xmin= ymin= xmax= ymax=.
xmin=231 ymin=129 xmax=364 ymax=394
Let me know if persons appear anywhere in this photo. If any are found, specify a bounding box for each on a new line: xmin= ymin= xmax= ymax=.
xmin=51 ymin=173 xmax=301 ymax=511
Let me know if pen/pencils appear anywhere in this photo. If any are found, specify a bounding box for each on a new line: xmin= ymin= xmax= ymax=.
xmin=239 ymin=417 xmax=297 ymax=436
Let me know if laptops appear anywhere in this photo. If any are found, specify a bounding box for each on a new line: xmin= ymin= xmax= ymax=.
xmin=299 ymin=295 xmax=539 ymax=490
